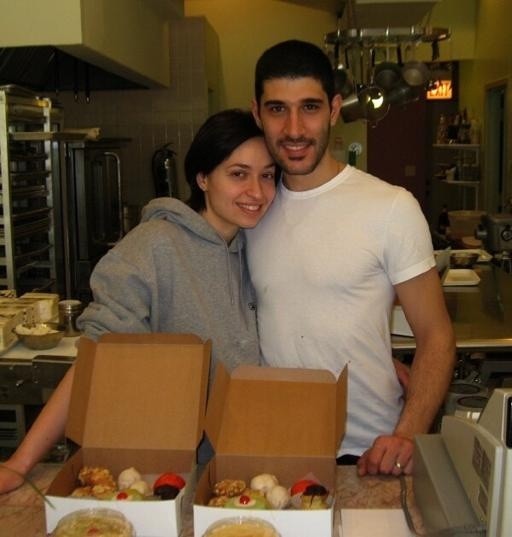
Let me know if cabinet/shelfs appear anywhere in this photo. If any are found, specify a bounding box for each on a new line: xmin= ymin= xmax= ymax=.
xmin=430 ymin=142 xmax=481 ymax=186
xmin=1 ymin=92 xmax=57 ymax=452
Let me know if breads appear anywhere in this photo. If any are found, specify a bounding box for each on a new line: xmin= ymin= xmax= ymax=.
xmin=68 ymin=464 xmax=184 ymax=500
xmin=206 ymin=472 xmax=330 ymax=510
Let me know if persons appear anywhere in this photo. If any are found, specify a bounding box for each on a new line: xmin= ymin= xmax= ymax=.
xmin=1 ymin=108 xmax=414 ymax=497
xmin=235 ymin=36 xmax=457 ymax=478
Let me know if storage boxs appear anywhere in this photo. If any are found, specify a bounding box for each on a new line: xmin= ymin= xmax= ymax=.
xmin=193 ymin=357 xmax=346 ymax=537
xmin=44 ymin=333 xmax=213 ymax=537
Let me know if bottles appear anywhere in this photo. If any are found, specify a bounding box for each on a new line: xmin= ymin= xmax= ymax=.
xmin=437 ymin=204 xmax=449 ymax=234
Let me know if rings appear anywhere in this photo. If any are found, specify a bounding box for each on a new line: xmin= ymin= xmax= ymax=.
xmin=394 ymin=461 xmax=404 ymax=469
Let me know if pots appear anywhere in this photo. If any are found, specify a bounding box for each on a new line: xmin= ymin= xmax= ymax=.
xmin=334 ymin=42 xmax=431 ymax=122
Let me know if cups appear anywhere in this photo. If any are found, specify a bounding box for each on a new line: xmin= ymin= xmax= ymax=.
xmin=59 ymin=300 xmax=81 ymax=336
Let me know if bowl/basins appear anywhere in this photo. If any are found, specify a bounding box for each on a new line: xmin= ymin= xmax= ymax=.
xmin=451 ymin=253 xmax=478 ymax=265
xmin=11 ymin=321 xmax=68 ymax=350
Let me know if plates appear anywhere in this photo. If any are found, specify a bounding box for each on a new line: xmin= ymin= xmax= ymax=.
xmin=443 ymin=268 xmax=481 ymax=286
